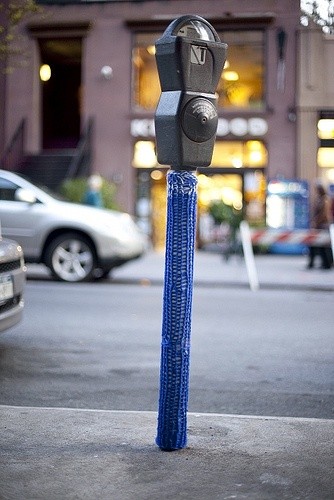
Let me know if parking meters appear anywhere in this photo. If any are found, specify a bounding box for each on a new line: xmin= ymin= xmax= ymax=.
xmin=152 ymin=13 xmax=228 ymax=451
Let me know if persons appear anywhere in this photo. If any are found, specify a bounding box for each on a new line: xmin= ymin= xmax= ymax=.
xmin=305 ymin=182 xmax=331 ymax=270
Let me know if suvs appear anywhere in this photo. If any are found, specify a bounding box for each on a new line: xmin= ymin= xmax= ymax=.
xmin=0 ymin=169 xmax=144 ymax=282
